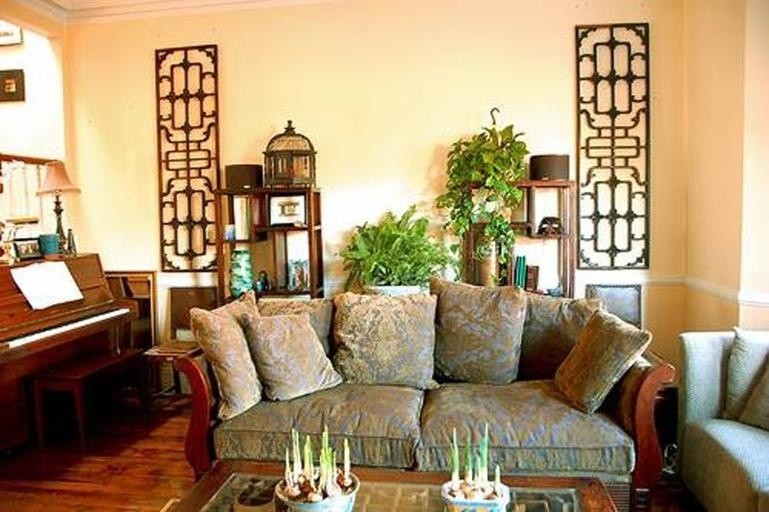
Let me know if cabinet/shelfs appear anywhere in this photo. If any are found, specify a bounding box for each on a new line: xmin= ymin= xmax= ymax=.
xmin=464 ymin=180 xmax=578 ymax=299
xmin=210 ymin=186 xmax=324 ymax=310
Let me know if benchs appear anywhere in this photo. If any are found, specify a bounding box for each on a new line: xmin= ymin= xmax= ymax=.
xmin=32 ymin=345 xmax=151 ymax=453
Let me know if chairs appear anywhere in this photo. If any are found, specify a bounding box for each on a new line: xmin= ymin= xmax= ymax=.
xmin=142 ymin=285 xmax=218 ymax=400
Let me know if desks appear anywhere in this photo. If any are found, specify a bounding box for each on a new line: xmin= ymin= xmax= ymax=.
xmin=169 ymin=457 xmax=621 ymax=511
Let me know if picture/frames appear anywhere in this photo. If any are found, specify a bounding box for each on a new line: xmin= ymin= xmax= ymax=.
xmin=1 ymin=22 xmax=25 ymax=47
xmin=12 ymin=237 xmax=45 ymax=263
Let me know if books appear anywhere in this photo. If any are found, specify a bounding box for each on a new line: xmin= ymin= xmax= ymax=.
xmin=9 ymin=259 xmax=84 ymax=310
xmin=514 ymin=254 xmax=538 ymax=292
xmin=143 ymin=338 xmax=201 ymax=357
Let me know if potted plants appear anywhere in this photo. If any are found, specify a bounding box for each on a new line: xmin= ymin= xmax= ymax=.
xmin=332 ymin=202 xmax=464 ymax=296
xmin=432 ymin=123 xmax=534 ymax=293
xmin=274 ymin=421 xmax=361 ymax=512
xmin=438 ymin=421 xmax=512 ymax=511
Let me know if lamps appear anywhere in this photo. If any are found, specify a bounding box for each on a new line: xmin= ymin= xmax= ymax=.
xmin=36 ymin=159 xmax=83 ymax=259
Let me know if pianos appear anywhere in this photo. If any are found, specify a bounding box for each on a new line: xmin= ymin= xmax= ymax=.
xmin=0 ymin=254 xmax=139 ymax=450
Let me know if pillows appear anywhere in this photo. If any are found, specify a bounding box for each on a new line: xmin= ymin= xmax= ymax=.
xmin=431 ymin=278 xmax=527 ymax=386
xmin=738 ymin=353 xmax=769 ymax=432
xmin=518 ymin=290 xmax=605 ymax=382
xmin=551 ymin=307 xmax=654 ymax=417
xmin=238 ymin=311 xmax=347 ymax=402
xmin=333 ymin=290 xmax=442 ymax=391
xmin=719 ymin=323 xmax=769 ymax=422
xmin=187 ymin=289 xmax=263 ymax=422
xmin=257 ymin=295 xmax=335 ymax=356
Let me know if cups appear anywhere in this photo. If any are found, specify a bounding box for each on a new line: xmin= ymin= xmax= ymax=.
xmin=39 ymin=234 xmax=60 ymax=260
xmin=547 ymin=288 xmax=566 ymax=296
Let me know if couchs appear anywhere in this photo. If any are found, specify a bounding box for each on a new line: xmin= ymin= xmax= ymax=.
xmin=676 ymin=328 xmax=769 ymax=511
xmin=169 ymin=294 xmax=677 ymax=511
